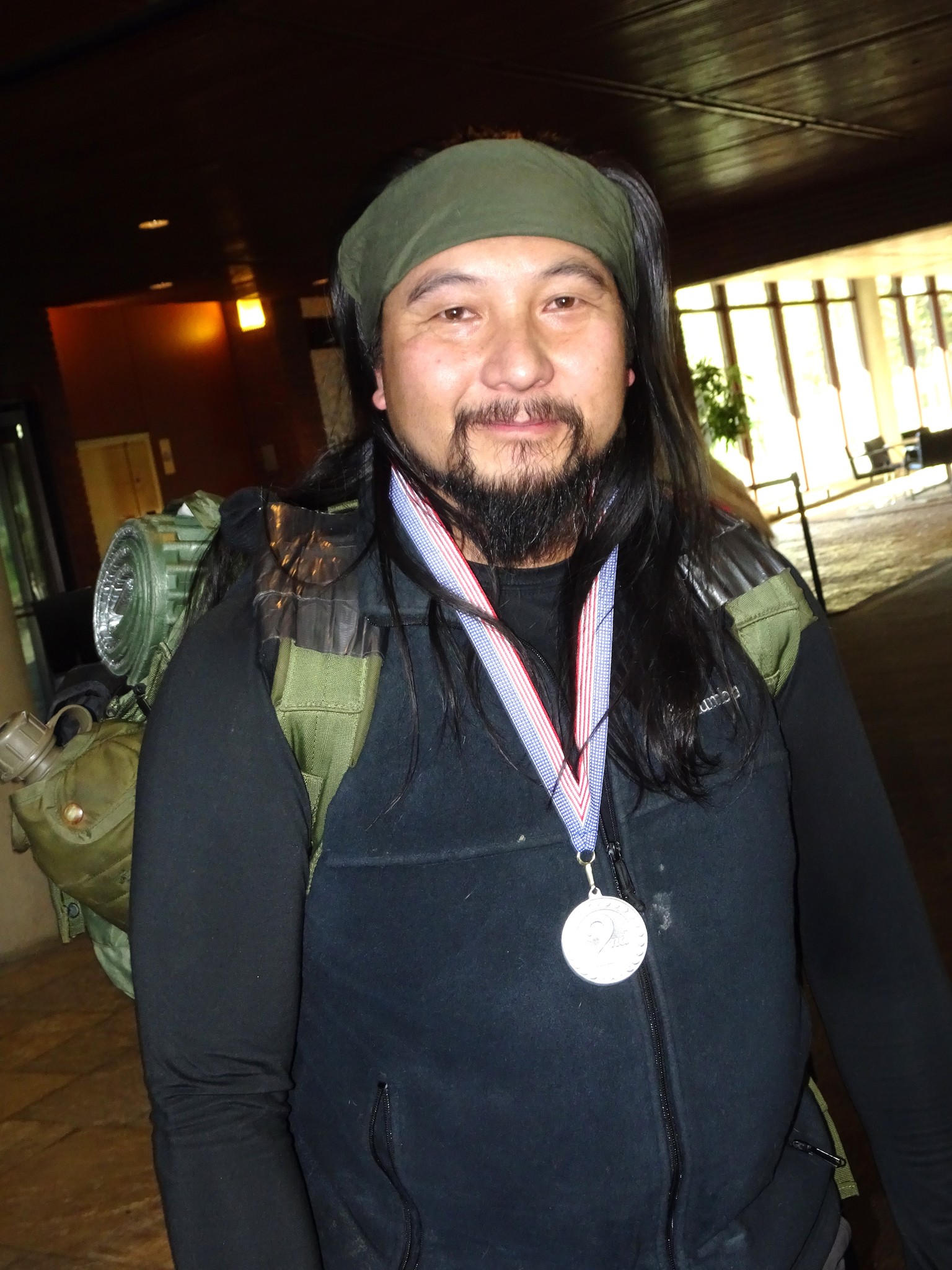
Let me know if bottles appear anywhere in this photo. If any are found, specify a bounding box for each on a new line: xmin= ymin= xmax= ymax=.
xmin=0 ymin=704 xmax=93 ymax=786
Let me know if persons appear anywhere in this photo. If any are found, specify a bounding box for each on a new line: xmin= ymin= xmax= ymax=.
xmin=128 ymin=139 xmax=952 ymax=1269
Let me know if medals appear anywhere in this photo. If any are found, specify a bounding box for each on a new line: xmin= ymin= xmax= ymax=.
xmin=561 ymin=885 xmax=648 ymax=986
